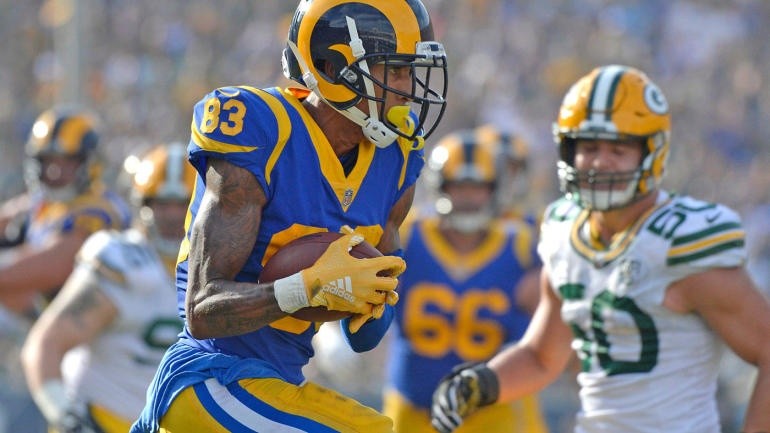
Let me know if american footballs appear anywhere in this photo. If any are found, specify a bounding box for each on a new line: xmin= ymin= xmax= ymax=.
xmin=256 ymin=232 xmax=389 ymax=320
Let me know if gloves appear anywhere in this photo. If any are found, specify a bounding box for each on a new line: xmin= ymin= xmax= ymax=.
xmin=429 ymin=361 xmax=499 ymax=433
xmin=275 ymin=235 xmax=406 ymax=335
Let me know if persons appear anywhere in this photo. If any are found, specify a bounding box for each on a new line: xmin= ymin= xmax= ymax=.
xmin=0 ymin=2 xmax=769 ymax=297
xmin=430 ymin=63 xmax=770 ymax=433
xmin=18 ymin=139 xmax=198 ymax=433
xmin=130 ymin=1 xmax=447 ymax=433
xmin=0 ymin=102 xmax=131 ymax=432
xmin=384 ymin=120 xmax=584 ymax=432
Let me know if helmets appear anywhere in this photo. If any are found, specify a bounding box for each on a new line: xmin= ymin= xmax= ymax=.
xmin=22 ymin=104 xmax=103 ymax=199
xmin=423 ymin=131 xmax=501 ymax=232
xmin=471 ymin=125 xmax=530 ymax=211
xmin=138 ymin=144 xmax=200 ymax=251
xmin=281 ymin=0 xmax=449 ymax=149
xmin=554 ymin=65 xmax=673 ymax=207
